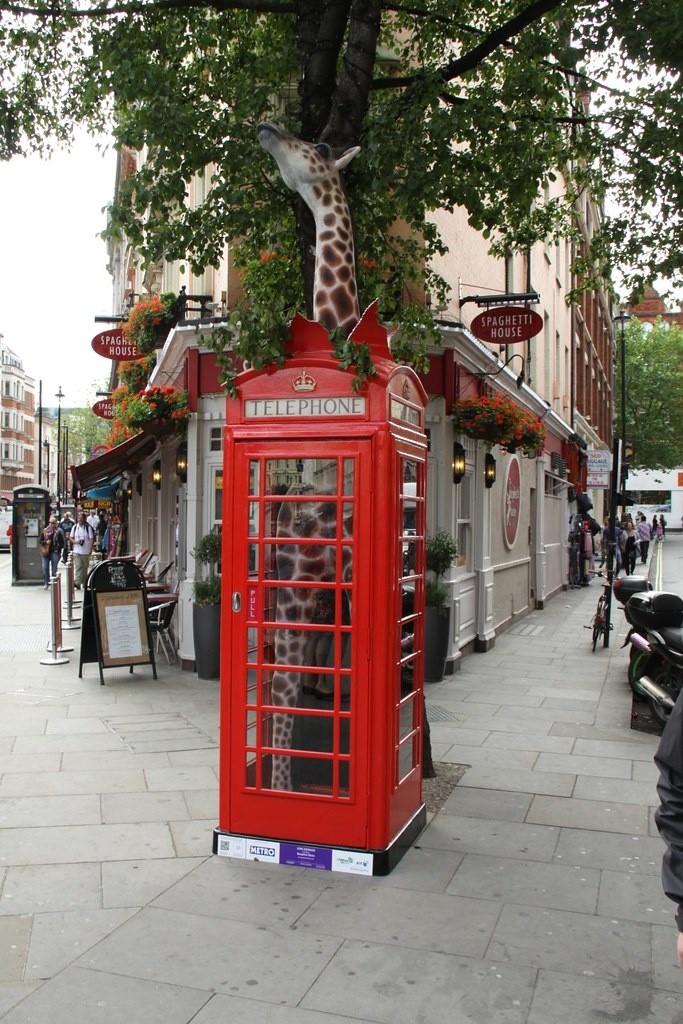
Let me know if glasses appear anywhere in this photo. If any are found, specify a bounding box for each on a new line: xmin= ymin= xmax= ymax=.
xmin=49 ymin=522 xmax=55 ymax=525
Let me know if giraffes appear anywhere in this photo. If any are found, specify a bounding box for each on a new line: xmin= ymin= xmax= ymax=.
xmin=258 ymin=120 xmax=360 ymax=792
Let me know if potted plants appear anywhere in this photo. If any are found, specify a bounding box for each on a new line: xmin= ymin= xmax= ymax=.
xmin=188 ymin=530 xmax=223 ymax=680
xmin=423 ymin=527 xmax=459 ymax=682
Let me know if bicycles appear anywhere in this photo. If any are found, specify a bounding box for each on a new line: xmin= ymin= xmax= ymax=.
xmin=583 ymin=567 xmax=620 ymax=653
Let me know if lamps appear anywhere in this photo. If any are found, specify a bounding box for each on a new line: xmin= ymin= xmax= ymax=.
xmin=152 ymin=459 xmax=161 ymax=490
xmin=126 ymin=304 xmax=133 ymax=308
xmin=135 ymin=473 xmax=142 ymax=496
xmin=454 ymin=441 xmax=465 ymax=484
xmin=129 ymin=293 xmax=138 ymax=297
xmin=206 ymin=302 xmax=219 ymax=318
xmin=485 ymin=453 xmax=496 ymax=488
xmin=176 ymin=441 xmax=187 ymax=484
xmin=469 ymin=354 xmax=525 ymax=390
xmin=435 ymin=305 xmax=448 ymax=318
xmin=127 ymin=481 xmax=132 ymax=500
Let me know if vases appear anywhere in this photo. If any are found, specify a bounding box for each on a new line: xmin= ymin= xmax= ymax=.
xmin=150 ymin=310 xmax=176 ymax=348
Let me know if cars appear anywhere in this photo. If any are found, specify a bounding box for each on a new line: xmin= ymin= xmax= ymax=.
xmin=0 ymin=497 xmax=15 ymax=555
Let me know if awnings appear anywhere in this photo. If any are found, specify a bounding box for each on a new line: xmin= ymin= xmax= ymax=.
xmin=69 ymin=430 xmax=158 ymax=508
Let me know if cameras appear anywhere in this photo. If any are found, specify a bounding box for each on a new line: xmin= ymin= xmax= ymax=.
xmin=79 ymin=539 xmax=84 ymax=546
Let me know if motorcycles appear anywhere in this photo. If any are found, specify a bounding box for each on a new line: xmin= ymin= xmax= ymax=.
xmin=612 ymin=574 xmax=683 ymax=727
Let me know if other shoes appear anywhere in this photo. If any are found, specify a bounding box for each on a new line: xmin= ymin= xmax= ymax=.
xmin=61 ymin=560 xmax=67 ymax=563
xmin=74 ymin=583 xmax=80 ymax=590
xmin=43 ymin=584 xmax=49 ymax=590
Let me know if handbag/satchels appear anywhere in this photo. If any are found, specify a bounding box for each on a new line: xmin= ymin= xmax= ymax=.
xmin=40 ymin=544 xmax=52 ymax=556
xmin=629 ymin=551 xmax=636 ymax=558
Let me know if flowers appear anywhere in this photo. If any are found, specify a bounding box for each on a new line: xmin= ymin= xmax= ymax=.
xmin=107 ymin=293 xmax=192 ymax=449
xmin=238 ymin=252 xmax=380 ymax=305
xmin=451 ymin=389 xmax=547 ymax=457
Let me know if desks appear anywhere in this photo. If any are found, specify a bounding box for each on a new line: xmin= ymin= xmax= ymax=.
xmin=109 ymin=556 xmax=178 ymax=602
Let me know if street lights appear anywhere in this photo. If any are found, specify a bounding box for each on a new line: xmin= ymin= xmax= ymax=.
xmin=35 ymin=406 xmax=44 ymax=484
xmin=61 ymin=418 xmax=69 ymax=505
xmin=602 ymin=310 xmax=632 ymax=647
xmin=55 ymin=385 xmax=65 ymax=502
xmin=43 ymin=439 xmax=51 ymax=490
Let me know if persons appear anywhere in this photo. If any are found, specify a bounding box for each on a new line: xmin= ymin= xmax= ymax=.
xmin=587 ymin=511 xmax=651 ymax=580
xmin=38 ymin=517 xmax=67 ymax=590
xmin=6 ymin=524 xmax=12 ymax=556
xmin=39 ymin=509 xmax=107 ymax=591
xmin=302 ymin=575 xmax=352 ymax=701
xmin=652 ymin=514 xmax=666 ymax=542
xmin=70 ymin=513 xmax=94 ymax=590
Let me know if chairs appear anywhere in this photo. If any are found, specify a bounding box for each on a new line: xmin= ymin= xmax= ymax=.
xmin=135 ymin=548 xmax=149 ymax=563
xmin=142 ymin=551 xmax=155 ymax=568
xmin=139 ymin=561 xmax=177 ymax=666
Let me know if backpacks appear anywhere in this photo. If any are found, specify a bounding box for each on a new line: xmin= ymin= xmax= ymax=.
xmin=587 ymin=514 xmax=600 ymax=536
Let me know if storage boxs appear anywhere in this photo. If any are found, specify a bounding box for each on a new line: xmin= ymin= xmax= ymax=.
xmin=613 ymin=576 xmax=653 ymax=604
xmin=624 ymin=591 xmax=683 ymax=632
